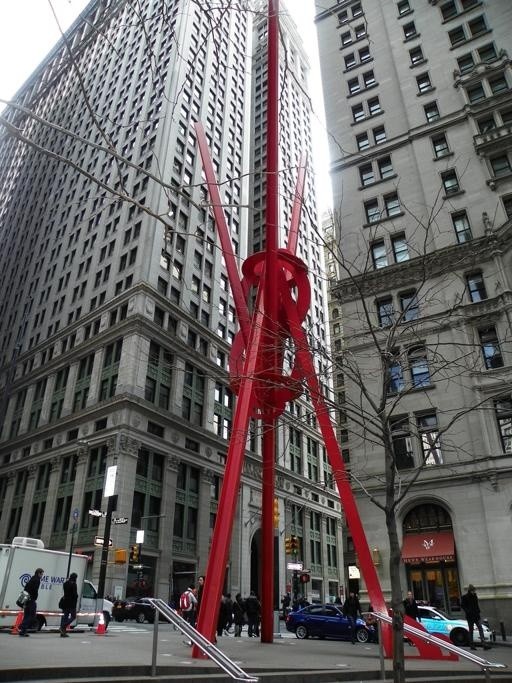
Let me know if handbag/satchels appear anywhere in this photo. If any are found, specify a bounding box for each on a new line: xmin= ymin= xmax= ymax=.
xmin=18 ymin=591 xmax=30 ymax=603
xmin=59 ymin=597 xmax=64 ymax=609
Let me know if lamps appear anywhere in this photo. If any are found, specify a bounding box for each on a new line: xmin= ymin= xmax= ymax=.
xmin=372 ymin=545 xmax=381 ymax=565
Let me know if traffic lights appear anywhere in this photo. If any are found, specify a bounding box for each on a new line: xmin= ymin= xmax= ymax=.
xmin=300 ymin=573 xmax=311 ymax=583
xmin=285 ymin=536 xmax=292 ymax=555
xmin=130 ymin=544 xmax=139 ymax=561
xmin=291 ymin=536 xmax=299 ymax=555
xmin=273 ymin=496 xmax=280 ymax=529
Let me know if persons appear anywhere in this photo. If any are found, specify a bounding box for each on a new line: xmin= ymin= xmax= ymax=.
xmin=460 ymin=584 xmax=492 ymax=651
xmin=58 ymin=572 xmax=78 ymax=637
xmin=281 ymin=593 xmax=310 ymax=620
xmin=402 ymin=591 xmax=420 ymax=646
xmin=180 ymin=576 xmax=261 ymax=637
xmin=15 ymin=568 xmax=44 ymax=637
xmin=343 ymin=589 xmax=361 ymax=645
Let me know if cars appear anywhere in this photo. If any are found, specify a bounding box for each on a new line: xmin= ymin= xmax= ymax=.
xmin=111 ymin=595 xmax=172 ymax=625
xmin=283 ymin=602 xmax=378 ymax=644
xmin=415 ymin=598 xmax=493 ymax=649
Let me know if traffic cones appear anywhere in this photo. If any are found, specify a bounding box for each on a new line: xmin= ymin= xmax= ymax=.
xmin=94 ymin=613 xmax=110 ymax=636
xmin=9 ymin=611 xmax=26 ymax=635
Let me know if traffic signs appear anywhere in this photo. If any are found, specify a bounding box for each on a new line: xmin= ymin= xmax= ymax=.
xmin=94 ymin=535 xmax=113 ymax=547
xmin=113 ymin=516 xmax=129 ymax=525
xmin=87 ymin=508 xmax=106 ymax=517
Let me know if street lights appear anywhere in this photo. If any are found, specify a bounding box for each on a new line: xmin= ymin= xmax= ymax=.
xmin=136 ymin=515 xmax=168 ymax=600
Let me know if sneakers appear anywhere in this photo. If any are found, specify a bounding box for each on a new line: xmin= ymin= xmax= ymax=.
xmin=470 ymin=644 xmax=476 ymax=650
xmin=224 ymin=630 xmax=257 ymax=637
xmin=483 ymin=643 xmax=492 ymax=649
xmin=59 ymin=626 xmax=69 ymax=637
xmin=16 ymin=624 xmax=29 ymax=637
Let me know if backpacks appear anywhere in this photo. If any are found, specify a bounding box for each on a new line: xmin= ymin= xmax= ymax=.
xmin=180 ymin=591 xmax=190 ymax=609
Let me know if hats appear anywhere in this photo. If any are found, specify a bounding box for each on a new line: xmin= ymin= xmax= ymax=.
xmin=349 ymin=588 xmax=355 ymax=593
xmin=468 ymin=584 xmax=476 ymax=592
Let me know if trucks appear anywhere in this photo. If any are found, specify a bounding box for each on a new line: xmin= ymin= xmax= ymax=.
xmin=0 ymin=534 xmax=117 ymax=632
